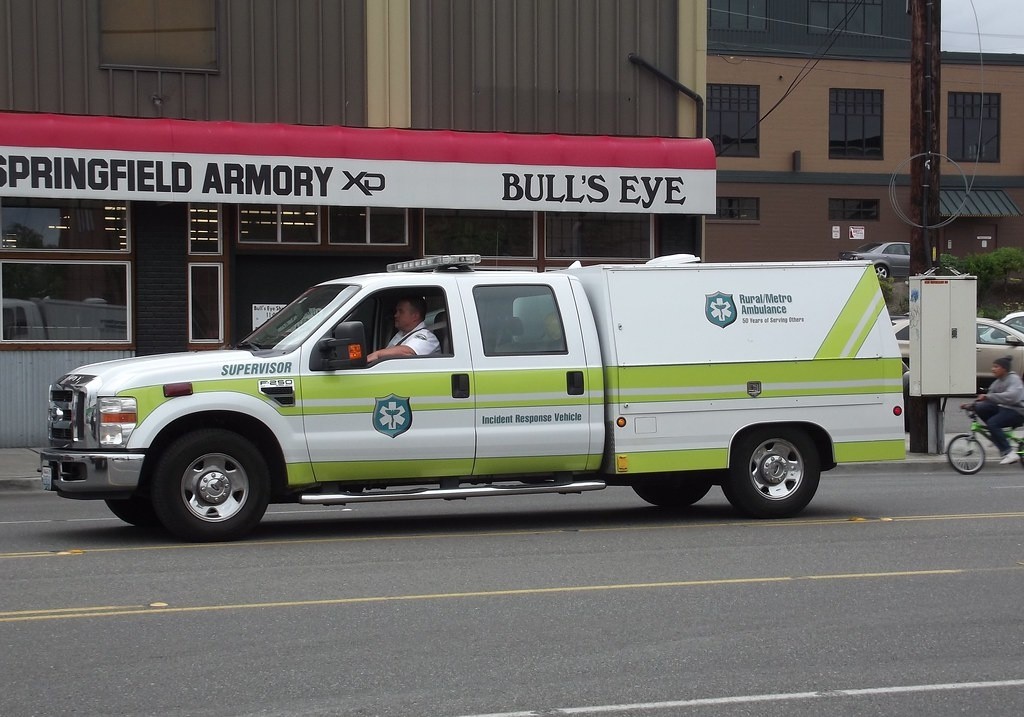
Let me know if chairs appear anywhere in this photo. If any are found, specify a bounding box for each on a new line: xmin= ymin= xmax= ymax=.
xmin=434 ymin=312 xmax=449 ymax=356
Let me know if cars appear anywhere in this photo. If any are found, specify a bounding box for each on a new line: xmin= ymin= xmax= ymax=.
xmin=891 ymin=313 xmax=1024 ymax=379
xmin=839 ymin=242 xmax=912 ymax=280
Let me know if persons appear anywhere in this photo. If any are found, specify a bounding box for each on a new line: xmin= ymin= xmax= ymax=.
xmin=365 ymin=292 xmax=442 ymax=363
xmin=960 ymin=355 xmax=1024 ymax=465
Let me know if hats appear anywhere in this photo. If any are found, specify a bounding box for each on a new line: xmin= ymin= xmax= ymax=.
xmin=994 ymin=355 xmax=1013 ymax=371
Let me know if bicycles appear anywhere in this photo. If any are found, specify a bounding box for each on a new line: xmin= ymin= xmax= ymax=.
xmin=945 ymin=386 xmax=1024 ymax=475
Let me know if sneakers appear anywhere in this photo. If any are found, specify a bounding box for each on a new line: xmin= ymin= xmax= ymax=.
xmin=999 ymin=454 xmax=1020 ymax=464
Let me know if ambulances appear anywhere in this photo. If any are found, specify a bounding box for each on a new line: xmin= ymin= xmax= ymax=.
xmin=35 ymin=254 xmax=917 ymax=544
